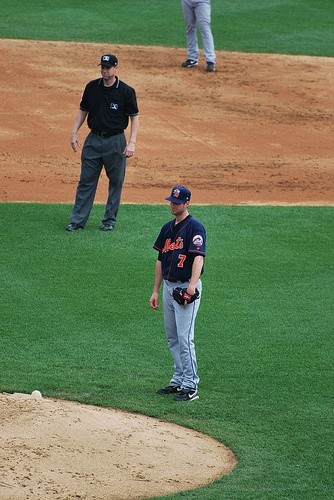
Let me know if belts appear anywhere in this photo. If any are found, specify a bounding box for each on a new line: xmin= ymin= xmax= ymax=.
xmin=168 ymin=278 xmax=189 ymax=283
xmin=90 ymin=129 xmax=121 ymax=139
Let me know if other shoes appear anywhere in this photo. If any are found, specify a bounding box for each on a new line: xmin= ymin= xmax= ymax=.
xmin=100 ymin=224 xmax=114 ymax=231
xmin=172 ymin=388 xmax=199 ymax=403
xmin=65 ymin=223 xmax=80 ymax=231
xmin=207 ymin=62 xmax=216 ymax=72
xmin=182 ymin=59 xmax=198 ymax=67
xmin=157 ymin=386 xmax=182 ymax=395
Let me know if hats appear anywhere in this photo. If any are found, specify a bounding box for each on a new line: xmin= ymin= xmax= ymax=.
xmin=165 ymin=186 xmax=191 ymax=204
xmin=97 ymin=54 xmax=118 ymax=68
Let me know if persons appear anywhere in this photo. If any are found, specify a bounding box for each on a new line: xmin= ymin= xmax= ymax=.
xmin=182 ymin=0 xmax=216 ymax=72
xmin=150 ymin=186 xmax=206 ymax=402
xmin=65 ymin=54 xmax=140 ymax=231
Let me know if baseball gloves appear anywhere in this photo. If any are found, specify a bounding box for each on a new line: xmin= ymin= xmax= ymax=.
xmin=172 ymin=287 xmax=199 ymax=306
